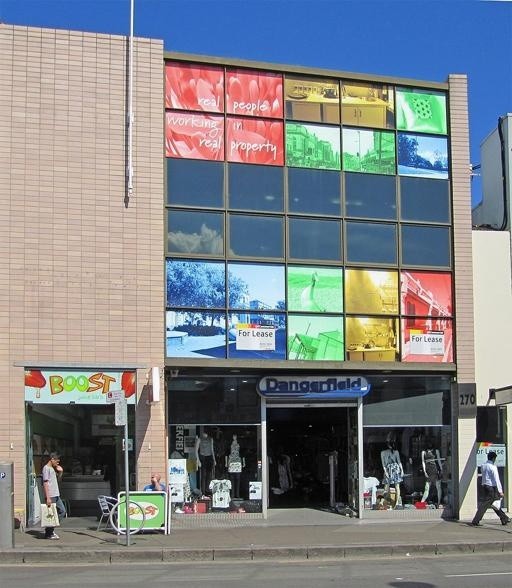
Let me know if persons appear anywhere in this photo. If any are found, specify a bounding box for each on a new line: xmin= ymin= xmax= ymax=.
xmin=41 ymin=451 xmax=66 ymax=540
xmin=194 ymin=432 xmax=217 ymax=495
xmin=421 ymin=448 xmax=442 ymax=506
xmin=380 ymin=441 xmax=405 ymax=510
xmin=471 ymin=451 xmax=511 ymax=526
xmin=225 ymin=432 xmax=246 ymax=498
xmin=142 ymin=473 xmax=165 ymax=496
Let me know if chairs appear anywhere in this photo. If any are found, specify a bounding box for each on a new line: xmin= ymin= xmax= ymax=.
xmin=96 ymin=495 xmax=118 ymax=532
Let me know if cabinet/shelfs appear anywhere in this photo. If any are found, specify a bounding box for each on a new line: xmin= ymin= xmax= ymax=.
xmin=349 ymin=351 xmax=363 ymax=361
xmin=323 ymin=103 xmax=386 ymax=128
xmin=364 ymin=351 xmax=395 ymax=362
xmin=292 ymin=102 xmax=320 ymax=121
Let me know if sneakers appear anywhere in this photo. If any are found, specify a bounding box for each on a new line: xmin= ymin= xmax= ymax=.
xmin=472 ymin=521 xmax=482 ymax=526
xmin=44 ymin=533 xmax=60 ymax=540
xmin=502 ymin=517 xmax=512 ymax=524
